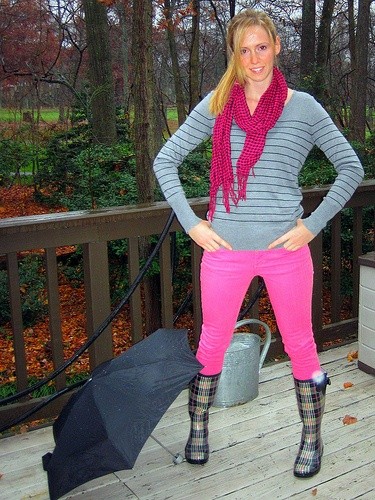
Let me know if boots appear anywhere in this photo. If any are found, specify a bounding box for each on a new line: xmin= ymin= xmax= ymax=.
xmin=184 ymin=370 xmax=331 ymax=480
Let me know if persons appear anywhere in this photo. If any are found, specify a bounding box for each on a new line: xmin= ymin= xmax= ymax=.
xmin=153 ymin=10 xmax=365 ymax=478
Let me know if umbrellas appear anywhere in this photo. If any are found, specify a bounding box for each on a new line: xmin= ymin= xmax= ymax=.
xmin=47 ymin=328 xmax=207 ymax=500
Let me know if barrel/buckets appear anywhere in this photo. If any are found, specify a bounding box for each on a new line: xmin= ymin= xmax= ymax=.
xmin=211 ymin=319 xmax=272 ymax=407
